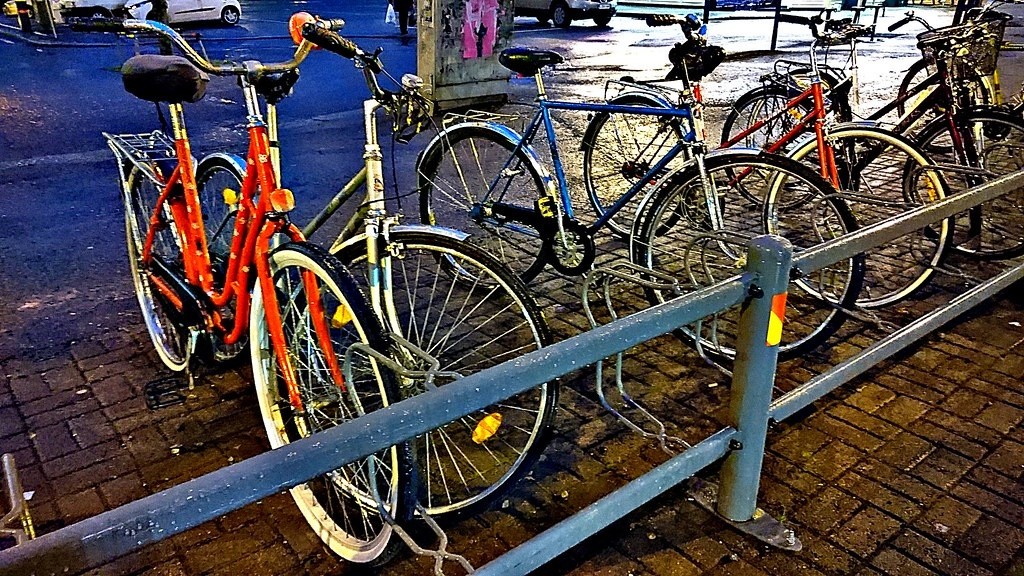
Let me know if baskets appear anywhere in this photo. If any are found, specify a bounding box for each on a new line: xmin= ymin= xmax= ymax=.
xmin=915 ymin=18 xmax=1005 ymax=80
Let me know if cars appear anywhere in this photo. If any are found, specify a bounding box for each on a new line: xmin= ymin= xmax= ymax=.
xmin=500 ymin=1 xmax=619 ymax=30
xmin=60 ymin=0 xmax=244 ymax=30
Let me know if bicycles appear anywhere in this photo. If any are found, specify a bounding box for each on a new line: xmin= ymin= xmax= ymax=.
xmin=191 ymin=23 xmax=557 ymax=526
xmin=102 ymin=14 xmax=414 ymax=571
xmin=412 ymin=0 xmax=1023 ymax=376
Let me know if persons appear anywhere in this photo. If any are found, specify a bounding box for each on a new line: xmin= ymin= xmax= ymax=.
xmin=388 ymin=0 xmax=416 ymax=34
